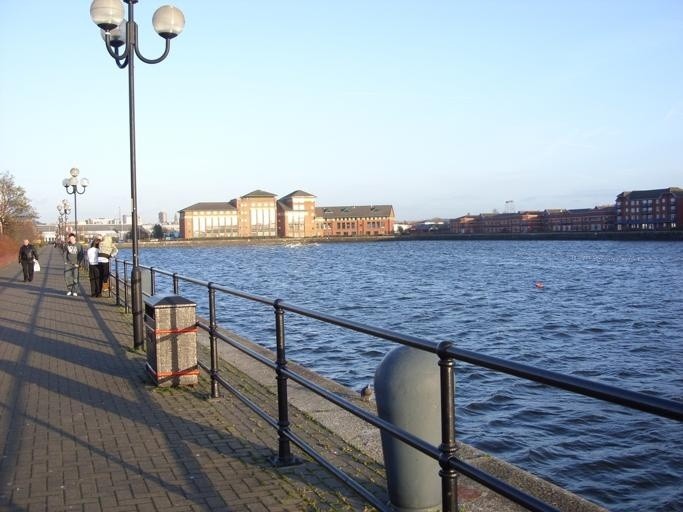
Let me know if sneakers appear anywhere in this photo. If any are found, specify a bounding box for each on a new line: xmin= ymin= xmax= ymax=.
xmin=67 ymin=291 xmax=77 ymax=296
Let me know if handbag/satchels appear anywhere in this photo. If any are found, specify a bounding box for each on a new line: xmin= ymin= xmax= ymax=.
xmin=32 ymin=258 xmax=40 ymax=272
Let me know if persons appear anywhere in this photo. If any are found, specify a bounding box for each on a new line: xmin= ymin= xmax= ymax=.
xmin=54 ymin=233 xmax=118 ymax=298
xmin=18 ymin=238 xmax=39 ymax=283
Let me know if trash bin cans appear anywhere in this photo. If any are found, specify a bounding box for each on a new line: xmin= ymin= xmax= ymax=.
xmin=142 ymin=294 xmax=199 ymax=386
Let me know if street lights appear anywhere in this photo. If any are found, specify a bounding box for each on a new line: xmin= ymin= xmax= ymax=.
xmin=55 ymin=200 xmax=72 ymax=243
xmin=63 ymin=168 xmax=88 ymax=241
xmin=90 ymin=0 xmax=185 ymax=350
